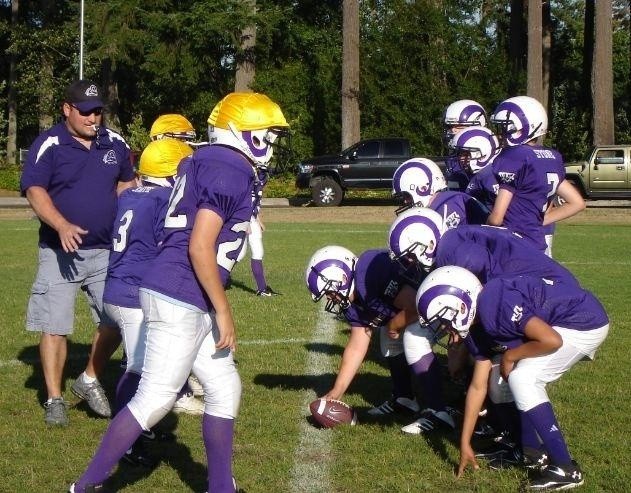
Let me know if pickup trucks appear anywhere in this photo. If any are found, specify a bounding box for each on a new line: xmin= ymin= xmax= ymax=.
xmin=296 ymin=138 xmax=449 ymax=207
xmin=554 ymin=145 xmax=631 ymax=207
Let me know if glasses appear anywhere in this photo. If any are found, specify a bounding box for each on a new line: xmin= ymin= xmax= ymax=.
xmin=70 ymin=104 xmax=104 ymax=116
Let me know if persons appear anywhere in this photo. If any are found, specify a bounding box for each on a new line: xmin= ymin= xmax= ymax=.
xmin=448 ymin=126 xmax=499 ymax=201
xmin=233 ymin=183 xmax=276 ymax=296
xmin=388 ymin=208 xmax=581 ymax=455
xmin=146 ymin=111 xmax=201 ymax=145
xmin=304 ymin=243 xmax=453 ymax=436
xmin=70 ymin=92 xmax=291 ymax=493
xmin=101 ymin=137 xmax=194 ymax=468
xmin=438 ymin=96 xmax=486 ymax=142
xmin=485 ymin=95 xmax=585 ymax=256
xmin=391 ymin=155 xmax=492 ymax=229
xmin=20 ymin=77 xmax=139 ymax=427
xmin=416 ymin=266 xmax=609 ymax=491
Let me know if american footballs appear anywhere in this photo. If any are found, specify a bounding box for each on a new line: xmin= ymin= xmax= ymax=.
xmin=309 ymin=398 xmax=360 ymax=429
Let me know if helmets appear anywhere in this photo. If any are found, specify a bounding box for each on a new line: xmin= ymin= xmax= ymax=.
xmin=207 ymin=92 xmax=292 ymax=179
xmin=449 ymin=127 xmax=501 ymax=176
xmin=306 ymin=245 xmax=360 ymax=313
xmin=415 ymin=266 xmax=484 ymax=349
xmin=392 ymin=157 xmax=447 ymax=213
xmin=489 ymin=96 xmax=548 ymax=151
xmin=139 ymin=139 xmax=195 ymax=188
xmin=388 ymin=206 xmax=449 ymax=284
xmin=150 ymin=114 xmax=196 ymax=141
xmin=445 ymin=100 xmax=486 ymax=148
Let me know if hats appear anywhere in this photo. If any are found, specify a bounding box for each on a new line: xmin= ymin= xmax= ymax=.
xmin=65 ymin=81 xmax=104 ymax=111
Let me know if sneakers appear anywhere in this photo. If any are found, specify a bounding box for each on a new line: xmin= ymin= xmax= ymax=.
xmin=526 ymin=461 xmax=584 ymax=492
xmin=138 ymin=430 xmax=177 ymax=443
xmin=366 ymin=395 xmax=420 ymax=415
xmin=489 ymin=446 xmax=548 ymax=470
xmin=122 ymin=444 xmax=155 ymax=469
xmin=256 ymin=286 xmax=284 ymax=297
xmin=474 ymin=432 xmax=515 ymax=457
xmin=474 ymin=419 xmax=501 ymax=437
xmin=189 ymin=376 xmax=204 ymax=396
xmin=173 ymin=392 xmax=205 ymax=416
xmin=70 ymin=374 xmax=112 ymax=418
xmin=401 ymin=409 xmax=456 ymax=434
xmin=43 ymin=397 xmax=72 ymax=427
